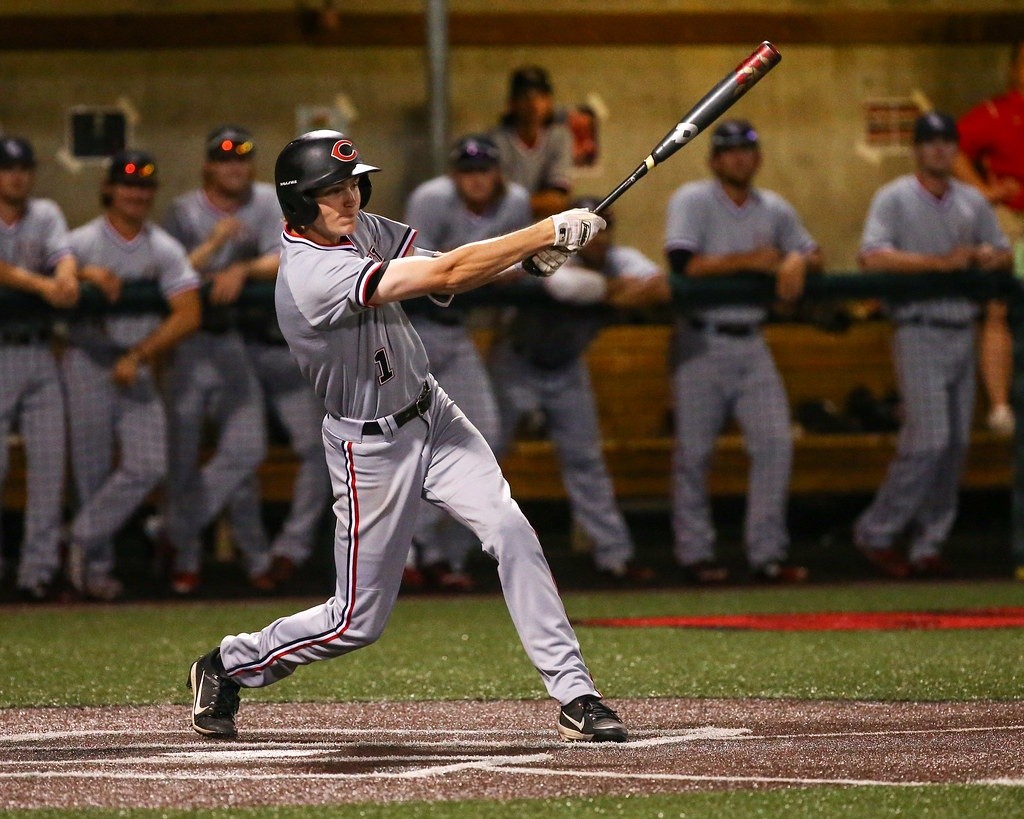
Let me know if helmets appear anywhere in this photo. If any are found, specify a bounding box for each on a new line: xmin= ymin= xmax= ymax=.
xmin=274 ymin=130 xmax=383 ymax=226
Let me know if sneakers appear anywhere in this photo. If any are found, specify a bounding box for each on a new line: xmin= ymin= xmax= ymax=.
xmin=186 ymin=646 xmax=242 ymax=739
xmin=557 ymin=694 xmax=628 ymax=743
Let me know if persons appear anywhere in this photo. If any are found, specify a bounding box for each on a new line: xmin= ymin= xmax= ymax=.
xmin=46 ymin=147 xmax=204 ymax=603
xmin=185 ymin=128 xmax=629 ymax=744
xmin=0 ymin=136 xmax=79 ymax=605
xmin=159 ymin=64 xmax=673 ymax=602
xmin=664 ymin=117 xmax=826 ymax=588
xmin=842 ymin=110 xmax=1014 ymax=581
xmin=946 ymin=39 xmax=1024 ymax=437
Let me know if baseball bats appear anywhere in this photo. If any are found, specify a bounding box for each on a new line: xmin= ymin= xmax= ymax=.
xmin=520 ymin=39 xmax=783 ymax=278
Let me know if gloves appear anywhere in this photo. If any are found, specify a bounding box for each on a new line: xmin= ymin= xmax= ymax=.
xmin=522 ymin=247 xmax=569 ymax=278
xmin=551 ymin=207 xmax=608 ymax=257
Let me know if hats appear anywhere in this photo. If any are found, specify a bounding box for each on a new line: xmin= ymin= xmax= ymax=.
xmin=204 ymin=126 xmax=264 ymax=161
xmin=449 ymin=133 xmax=502 ymax=173
xmin=108 ymin=149 xmax=160 ymax=188
xmin=913 ymin=113 xmax=959 ymax=145
xmin=0 ymin=135 xmax=37 ymax=171
xmin=507 ymin=64 xmax=554 ymax=99
xmin=711 ymin=119 xmax=761 ymax=149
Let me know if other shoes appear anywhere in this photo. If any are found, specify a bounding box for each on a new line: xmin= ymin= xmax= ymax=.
xmin=6 ymin=548 xmax=1024 ymax=606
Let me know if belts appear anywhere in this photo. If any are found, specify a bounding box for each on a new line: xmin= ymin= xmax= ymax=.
xmin=327 ymin=380 xmax=432 ymax=435
xmin=907 ymin=313 xmax=971 ymax=330
xmin=685 ymin=316 xmax=754 ymax=338
